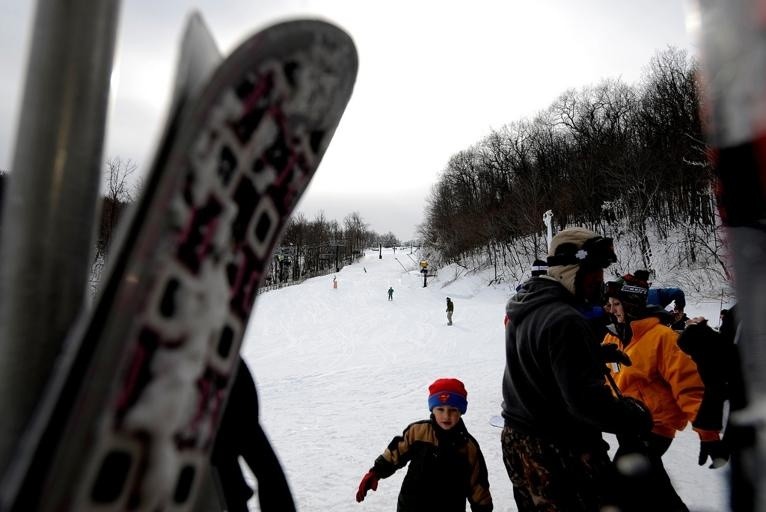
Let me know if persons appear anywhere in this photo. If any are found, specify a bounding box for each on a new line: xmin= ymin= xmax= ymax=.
xmin=445 ymin=296 xmax=454 ymax=327
xmin=355 ymin=378 xmax=493 ymax=512
xmin=504 ymin=259 xmax=547 ymax=331
xmin=601 ymin=270 xmax=753 ymax=512
xmin=387 ymin=286 xmax=395 ymax=300
xmin=500 ymin=226 xmax=650 ymax=512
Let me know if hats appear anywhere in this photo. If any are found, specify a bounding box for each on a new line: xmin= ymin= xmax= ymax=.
xmin=428 ymin=378 xmax=468 ymax=414
xmin=531 ymin=259 xmax=547 ymax=276
xmin=608 ymin=270 xmax=650 ymax=321
xmin=547 ymin=228 xmax=618 ymax=299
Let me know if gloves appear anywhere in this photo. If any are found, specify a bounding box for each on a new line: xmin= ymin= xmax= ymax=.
xmin=356 ymin=470 xmax=378 ymax=503
xmin=670 ymin=305 xmax=683 ymax=324
xmin=698 ymin=441 xmax=729 ymax=468
xmin=603 ymin=342 xmax=631 ymax=366
xmin=603 ymin=397 xmax=653 ymax=435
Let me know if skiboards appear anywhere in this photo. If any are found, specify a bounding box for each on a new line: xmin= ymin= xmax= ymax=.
xmin=1 ymin=0 xmax=359 ymax=512
